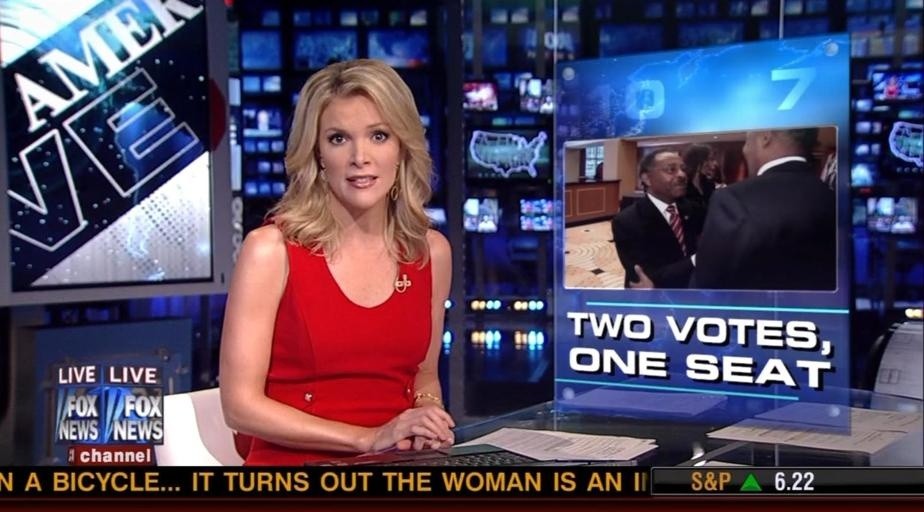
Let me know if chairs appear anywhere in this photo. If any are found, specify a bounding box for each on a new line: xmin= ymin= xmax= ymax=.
xmin=150 ymin=387 xmax=244 ymax=470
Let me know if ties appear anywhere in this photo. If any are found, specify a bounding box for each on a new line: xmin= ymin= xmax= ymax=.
xmin=667 ymin=206 xmax=686 ymax=256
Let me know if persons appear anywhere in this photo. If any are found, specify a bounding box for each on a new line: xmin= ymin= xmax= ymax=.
xmin=612 ymin=127 xmax=836 ymax=290
xmin=220 ymin=59 xmax=455 ymax=465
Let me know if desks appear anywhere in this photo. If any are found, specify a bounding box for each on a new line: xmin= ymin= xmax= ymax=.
xmin=307 ymin=366 xmax=919 ymax=468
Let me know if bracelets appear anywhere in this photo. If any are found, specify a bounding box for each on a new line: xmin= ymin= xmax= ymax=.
xmin=410 ymin=392 xmax=439 ymax=404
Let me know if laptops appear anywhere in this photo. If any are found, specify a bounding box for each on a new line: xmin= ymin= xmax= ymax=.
xmin=304 ymin=444 xmax=638 ymax=466
